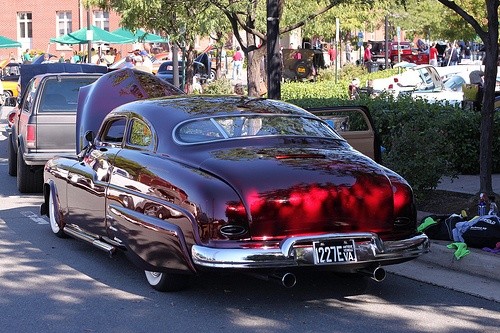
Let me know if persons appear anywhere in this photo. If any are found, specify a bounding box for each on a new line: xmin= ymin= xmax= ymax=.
xmin=348 ymin=84 xmax=360 ymax=100
xmin=328 ymin=45 xmax=336 ymax=61
xmin=447 ymin=43 xmax=457 ymax=66
xmin=9 ymin=52 xmax=15 ymax=60
xmin=59 ymin=54 xmax=65 ymax=63
xmin=444 ymin=43 xmax=450 ymax=65
xmin=34 ymin=51 xmax=37 ymax=57
xmin=24 ymin=48 xmax=30 ymax=61
xmin=455 ymin=42 xmax=461 ymax=62
xmin=72 ymin=44 xmax=165 ymax=64
xmin=430 ymin=43 xmax=439 ymax=67
xmin=364 ymin=43 xmax=372 ymax=60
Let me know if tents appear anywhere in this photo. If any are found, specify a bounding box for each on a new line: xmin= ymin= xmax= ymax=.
xmin=0 ymin=35 xmax=22 ymax=61
xmin=46 ymin=24 xmax=134 ymax=63
xmin=111 ymin=25 xmax=173 ymax=61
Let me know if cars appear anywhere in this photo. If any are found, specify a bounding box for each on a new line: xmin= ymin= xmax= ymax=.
xmin=433 ymin=40 xmax=484 ymax=66
xmin=39 ymin=67 xmax=431 ymax=293
xmin=7 ymin=62 xmax=118 ymax=195
xmin=152 ymin=61 xmax=206 ymax=92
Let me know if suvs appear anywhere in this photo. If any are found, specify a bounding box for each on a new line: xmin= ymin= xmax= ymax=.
xmin=368 ymin=41 xmax=430 ymax=72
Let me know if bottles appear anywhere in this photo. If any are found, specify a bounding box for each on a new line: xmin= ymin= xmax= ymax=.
xmin=461 ymin=209 xmax=468 ymax=218
xmin=477 ymin=192 xmax=498 ymax=216
